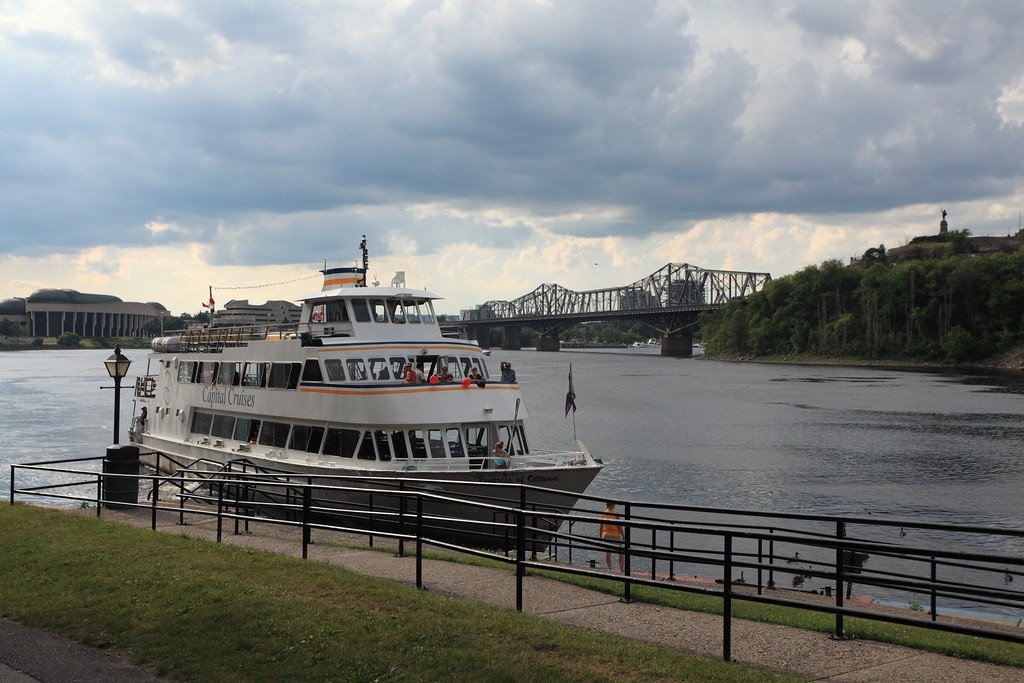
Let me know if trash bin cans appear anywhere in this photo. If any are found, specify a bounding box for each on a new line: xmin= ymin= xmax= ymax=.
xmin=101 ymin=445 xmax=141 ymax=509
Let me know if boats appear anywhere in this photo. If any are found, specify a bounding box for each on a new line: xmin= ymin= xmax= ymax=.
xmin=127 ymin=233 xmax=604 ymax=558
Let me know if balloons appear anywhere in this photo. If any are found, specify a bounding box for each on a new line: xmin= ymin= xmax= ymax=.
xmin=463 ymin=378 xmax=471 ymax=387
xmin=430 ymin=375 xmax=438 ymax=384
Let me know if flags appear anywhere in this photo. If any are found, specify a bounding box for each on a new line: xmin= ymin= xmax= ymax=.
xmin=565 ymin=363 xmax=576 ymax=419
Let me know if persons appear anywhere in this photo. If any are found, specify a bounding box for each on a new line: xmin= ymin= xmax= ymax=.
xmin=493 ymin=441 xmax=510 ymax=469
xmin=501 ymin=361 xmax=516 ymax=382
xmin=249 ymin=424 xmax=260 ymax=444
xmin=469 ymin=367 xmax=482 ymax=379
xmin=403 ymin=363 xmax=417 ymax=384
xmin=140 ymin=406 xmax=147 ymax=426
xmin=439 ymin=367 xmax=450 ymax=382
xmin=600 ymin=502 xmax=625 ymax=572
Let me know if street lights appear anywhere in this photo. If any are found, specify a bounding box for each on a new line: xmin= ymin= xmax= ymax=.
xmin=100 ymin=343 xmax=136 ymax=446
